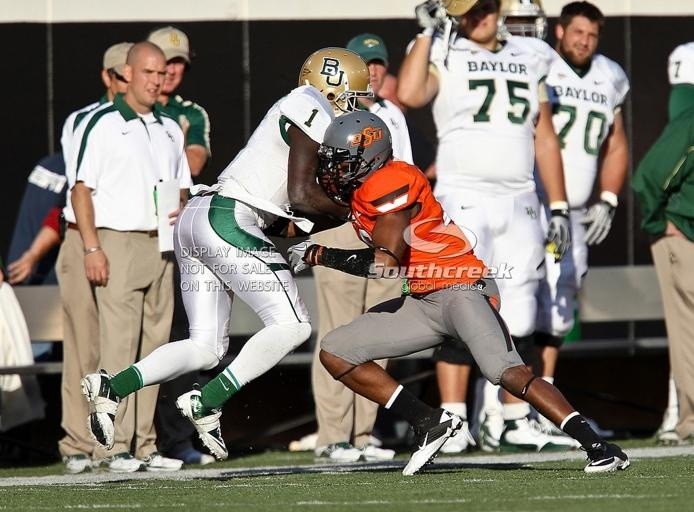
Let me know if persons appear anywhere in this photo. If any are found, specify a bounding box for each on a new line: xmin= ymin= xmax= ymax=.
xmin=290 ymin=72 xmax=436 ymax=452
xmin=288 ymin=110 xmax=630 ymax=476
xmin=311 ymin=33 xmax=415 ymax=463
xmin=396 ymin=0 xmax=571 ymax=458
xmin=498 ymin=0 xmax=550 ymax=42
xmin=530 ymin=1 xmax=632 ymax=440
xmin=2 ymin=22 xmax=214 ymax=475
xmin=631 ymin=41 xmax=694 ymax=447
xmin=81 ymin=47 xmax=376 ymax=460
xmin=148 ymin=25 xmax=217 ymax=468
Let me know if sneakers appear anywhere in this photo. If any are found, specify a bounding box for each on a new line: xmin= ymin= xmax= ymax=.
xmin=63 ymin=441 xmax=217 ymax=475
xmin=656 ymin=431 xmax=693 ymax=445
xmin=357 ymin=443 xmax=395 ymax=460
xmin=439 ymin=420 xmax=476 ymax=453
xmin=81 ymin=370 xmax=120 ymax=448
xmin=175 ymin=383 xmax=228 ymax=461
xmin=402 ymin=407 xmax=464 ymax=475
xmin=499 ymin=415 xmax=580 ymax=455
xmin=315 ymin=443 xmax=365 ymax=463
xmin=581 ymin=440 xmax=629 ymax=473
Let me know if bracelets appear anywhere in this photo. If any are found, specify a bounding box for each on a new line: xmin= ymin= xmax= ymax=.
xmin=317 ymin=245 xmax=375 ymax=279
xmin=82 ymin=246 xmax=100 ymax=255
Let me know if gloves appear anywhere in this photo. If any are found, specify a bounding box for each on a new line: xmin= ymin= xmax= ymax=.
xmin=579 ymin=191 xmax=618 ymax=245
xmin=287 ymin=241 xmax=328 ymax=277
xmin=547 ymin=201 xmax=572 ymax=263
xmin=416 ymin=1 xmax=445 ymax=37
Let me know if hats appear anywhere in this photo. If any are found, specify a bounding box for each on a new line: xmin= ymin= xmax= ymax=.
xmin=347 ymin=34 xmax=389 ymax=66
xmin=103 ymin=42 xmax=133 ymax=76
xmin=148 ymin=26 xmax=189 ymax=64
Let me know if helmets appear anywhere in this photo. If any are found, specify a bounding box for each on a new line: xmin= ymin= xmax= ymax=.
xmin=299 ymin=47 xmax=374 ymax=119
xmin=317 ymin=111 xmax=393 ymax=207
xmin=496 ymin=1 xmax=548 ymax=45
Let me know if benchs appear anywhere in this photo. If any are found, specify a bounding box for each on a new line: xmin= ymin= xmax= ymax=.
xmin=1 ymin=265 xmax=672 ymax=438
